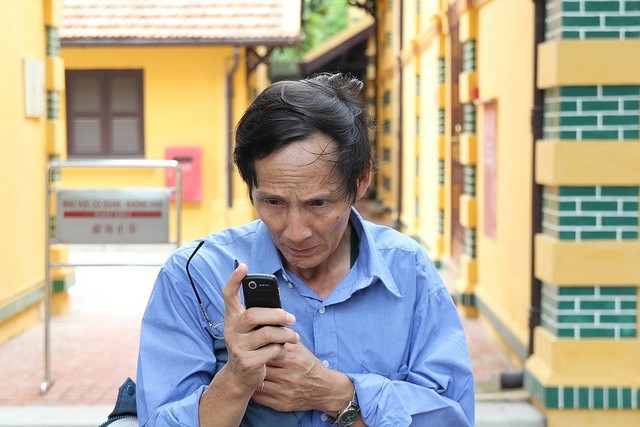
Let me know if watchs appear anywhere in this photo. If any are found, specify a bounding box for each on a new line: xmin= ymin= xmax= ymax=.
xmin=330 ymin=389 xmax=361 ymax=427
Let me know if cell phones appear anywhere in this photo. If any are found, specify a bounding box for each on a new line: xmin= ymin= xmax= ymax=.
xmin=241 ymin=273 xmax=286 ymax=348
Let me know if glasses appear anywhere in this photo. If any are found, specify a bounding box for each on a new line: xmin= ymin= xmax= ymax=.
xmin=186 ymin=241 xmax=239 ymax=339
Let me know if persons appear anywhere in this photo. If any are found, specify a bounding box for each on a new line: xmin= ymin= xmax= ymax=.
xmin=136 ymin=70 xmax=474 ymax=427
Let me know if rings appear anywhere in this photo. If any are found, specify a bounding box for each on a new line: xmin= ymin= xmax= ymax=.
xmin=258 ymin=382 xmax=266 ymax=394
